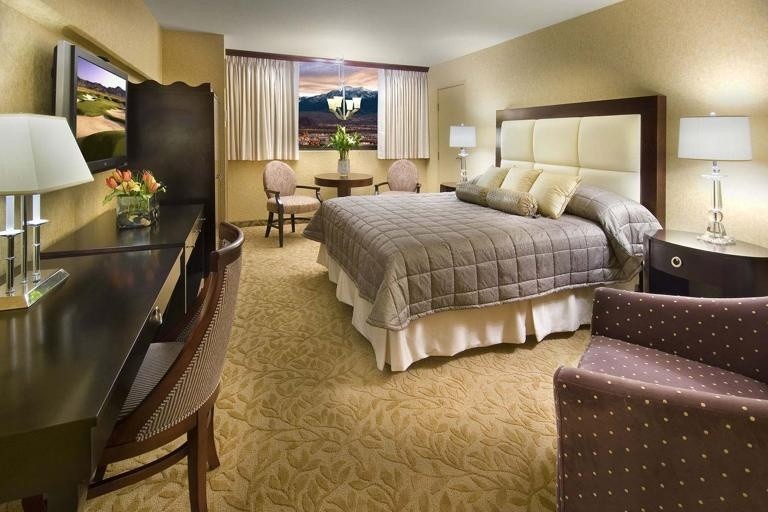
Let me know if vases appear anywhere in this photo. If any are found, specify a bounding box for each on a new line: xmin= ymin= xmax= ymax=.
xmin=114 ymin=195 xmax=160 ymax=230
xmin=337 ymin=147 xmax=350 ymax=176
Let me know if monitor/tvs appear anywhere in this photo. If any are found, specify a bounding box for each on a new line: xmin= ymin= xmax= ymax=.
xmin=50 ymin=40 xmax=131 ymax=176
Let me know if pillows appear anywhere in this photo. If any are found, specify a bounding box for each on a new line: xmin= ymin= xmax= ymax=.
xmin=456 ymin=115 xmax=640 ymax=219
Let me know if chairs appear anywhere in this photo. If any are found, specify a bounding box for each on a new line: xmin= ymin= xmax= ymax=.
xmin=263 ymin=160 xmax=323 ymax=247
xmin=374 ymin=159 xmax=422 ymax=196
xmin=553 ymin=285 xmax=768 ymax=512
xmin=86 ymin=220 xmax=246 ymax=512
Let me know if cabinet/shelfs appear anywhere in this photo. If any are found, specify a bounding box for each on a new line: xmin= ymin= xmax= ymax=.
xmin=128 ymin=80 xmax=219 ymax=276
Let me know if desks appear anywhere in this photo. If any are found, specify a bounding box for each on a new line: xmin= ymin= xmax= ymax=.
xmin=0 ymin=247 xmax=185 ymax=512
xmin=314 ymin=173 xmax=373 ymax=197
xmin=41 ymin=203 xmax=207 ymax=267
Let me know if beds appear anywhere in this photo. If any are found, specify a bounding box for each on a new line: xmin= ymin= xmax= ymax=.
xmin=301 ymin=95 xmax=667 ymax=372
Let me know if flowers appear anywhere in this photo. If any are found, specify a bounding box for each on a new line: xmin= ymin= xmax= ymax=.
xmin=102 ymin=169 xmax=166 ymax=212
xmin=327 ymin=124 xmax=362 ymax=161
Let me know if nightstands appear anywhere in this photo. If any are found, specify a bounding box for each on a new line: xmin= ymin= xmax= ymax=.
xmin=642 ymin=229 xmax=768 ymax=298
xmin=440 ymin=182 xmax=457 ymax=192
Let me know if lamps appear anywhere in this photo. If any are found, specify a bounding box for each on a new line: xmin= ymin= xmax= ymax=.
xmin=449 ymin=123 xmax=476 ymax=185
xmin=0 ymin=113 xmax=95 ymax=312
xmin=677 ymin=116 xmax=753 ymax=247
xmin=327 ymin=58 xmax=363 ymax=121
